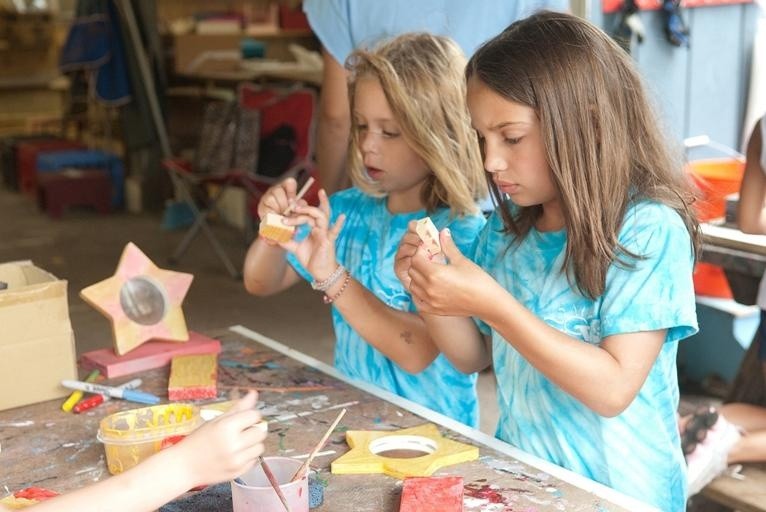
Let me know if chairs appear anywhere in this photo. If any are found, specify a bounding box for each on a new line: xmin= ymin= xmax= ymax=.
xmin=161 ymin=82 xmax=319 ymax=281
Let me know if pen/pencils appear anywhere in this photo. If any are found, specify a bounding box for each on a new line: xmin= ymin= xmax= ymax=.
xmin=282 ymin=175 xmax=317 ymax=217
xmin=60 ymin=370 xmax=159 ymax=413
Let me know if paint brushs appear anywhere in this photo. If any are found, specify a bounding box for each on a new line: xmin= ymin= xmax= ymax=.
xmin=290 ymin=408 xmax=347 ymax=482
xmin=258 ymin=455 xmax=292 ymax=512
xmin=249 ymin=401 xmax=361 ymax=429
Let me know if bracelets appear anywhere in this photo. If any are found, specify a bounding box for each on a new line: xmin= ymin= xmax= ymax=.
xmin=311 ymin=264 xmax=351 ymax=305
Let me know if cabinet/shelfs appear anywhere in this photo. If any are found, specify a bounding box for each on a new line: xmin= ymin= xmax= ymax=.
xmin=0 ymin=0 xmax=66 ymax=91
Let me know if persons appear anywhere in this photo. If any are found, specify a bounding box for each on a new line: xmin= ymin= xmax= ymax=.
xmin=394 ymin=11 xmax=701 ymax=511
xmin=16 ymin=387 xmax=269 ymax=512
xmin=243 ymin=32 xmax=489 ymax=431
xmin=316 ymin=41 xmax=351 ymax=205
xmin=678 ymin=111 xmax=766 ymax=501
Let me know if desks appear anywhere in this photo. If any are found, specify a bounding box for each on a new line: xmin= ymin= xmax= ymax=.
xmin=692 ymin=217 xmax=766 ymax=279
xmin=0 ymin=322 xmax=648 ymax=512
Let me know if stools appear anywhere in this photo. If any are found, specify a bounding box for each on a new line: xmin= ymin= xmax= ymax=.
xmin=15 ymin=138 xmax=87 ymax=194
xmin=34 ymin=169 xmax=112 ymax=219
xmin=694 ymin=463 xmax=764 ymax=511
xmin=680 ymin=296 xmax=763 ymax=390
xmin=33 ymin=149 xmax=126 ymax=215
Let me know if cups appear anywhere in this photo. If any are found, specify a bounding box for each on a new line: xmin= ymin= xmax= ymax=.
xmin=230 ymin=454 xmax=310 ymax=512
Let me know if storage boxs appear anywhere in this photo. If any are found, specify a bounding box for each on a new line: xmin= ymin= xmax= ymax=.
xmin=0 ymin=256 xmax=80 ymax=416
